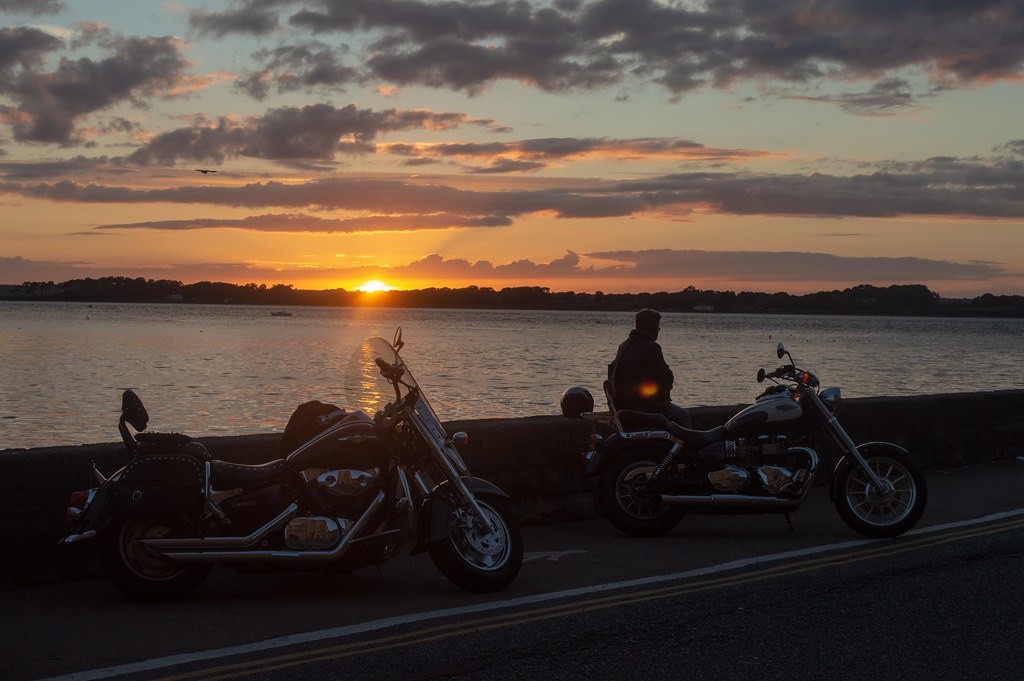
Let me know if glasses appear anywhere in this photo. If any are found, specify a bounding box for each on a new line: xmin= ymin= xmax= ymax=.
xmin=655 ymin=327 xmax=661 ymax=331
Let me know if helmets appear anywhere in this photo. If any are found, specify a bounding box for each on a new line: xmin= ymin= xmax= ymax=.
xmin=560 ymin=386 xmax=594 ymax=418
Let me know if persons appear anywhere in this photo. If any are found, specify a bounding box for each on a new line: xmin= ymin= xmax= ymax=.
xmin=612 ymin=309 xmax=691 ymax=429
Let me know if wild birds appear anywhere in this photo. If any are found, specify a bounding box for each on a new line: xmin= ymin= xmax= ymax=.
xmin=87 ymin=316 xmax=90 ymax=319
xmin=195 ymin=168 xmax=217 ymax=174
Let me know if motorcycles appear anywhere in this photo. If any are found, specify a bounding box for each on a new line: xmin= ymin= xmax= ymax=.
xmin=56 ymin=324 xmax=523 ymax=593
xmin=559 ymin=342 xmax=928 ymax=537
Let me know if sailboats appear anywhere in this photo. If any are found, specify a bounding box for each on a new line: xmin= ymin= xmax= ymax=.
xmin=270 ymin=284 xmax=292 ymax=317
